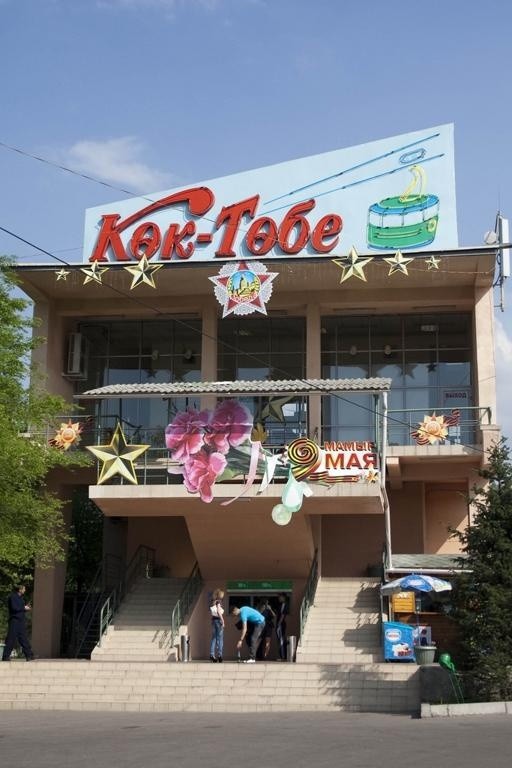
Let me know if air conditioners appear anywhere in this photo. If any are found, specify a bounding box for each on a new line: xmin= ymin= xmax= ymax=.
xmin=62 ymin=332 xmax=90 ymax=381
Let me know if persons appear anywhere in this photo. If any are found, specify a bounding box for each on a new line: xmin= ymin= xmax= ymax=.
xmin=1 ymin=583 xmax=39 ymax=661
xmin=253 ymin=597 xmax=277 ymax=660
xmin=208 ymin=588 xmax=225 ymax=663
xmin=229 ymin=604 xmax=265 ymax=663
xmin=275 ymin=592 xmax=290 ymax=661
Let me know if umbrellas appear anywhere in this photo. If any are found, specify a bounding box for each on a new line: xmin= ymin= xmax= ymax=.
xmin=378 ymin=571 xmax=452 ymax=644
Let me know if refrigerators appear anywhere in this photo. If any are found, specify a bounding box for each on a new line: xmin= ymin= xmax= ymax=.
xmin=383 ymin=621 xmax=416 ymax=661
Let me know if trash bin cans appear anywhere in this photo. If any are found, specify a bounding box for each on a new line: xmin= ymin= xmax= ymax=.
xmin=287 ymin=636 xmax=297 ymax=662
xmin=181 ymin=635 xmax=190 ymax=660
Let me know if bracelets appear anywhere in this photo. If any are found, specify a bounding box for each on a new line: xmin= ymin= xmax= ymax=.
xmin=239 ymin=639 xmax=243 ymax=641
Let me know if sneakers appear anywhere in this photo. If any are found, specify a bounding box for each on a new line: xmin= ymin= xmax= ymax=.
xmin=26 ymin=654 xmax=38 ymax=660
xmin=244 ymin=658 xmax=255 ymax=663
xmin=2 ymin=657 xmax=10 ymax=661
xmin=262 ymin=656 xmax=287 ymax=662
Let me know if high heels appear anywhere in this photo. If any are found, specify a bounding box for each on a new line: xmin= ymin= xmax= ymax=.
xmin=210 ymin=656 xmax=217 ymax=662
xmin=219 ymin=657 xmax=222 ymax=662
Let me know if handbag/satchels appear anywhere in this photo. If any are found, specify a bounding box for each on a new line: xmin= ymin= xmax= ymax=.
xmin=262 ymin=608 xmax=276 ymax=618
xmin=210 ymin=605 xmax=224 ymax=615
